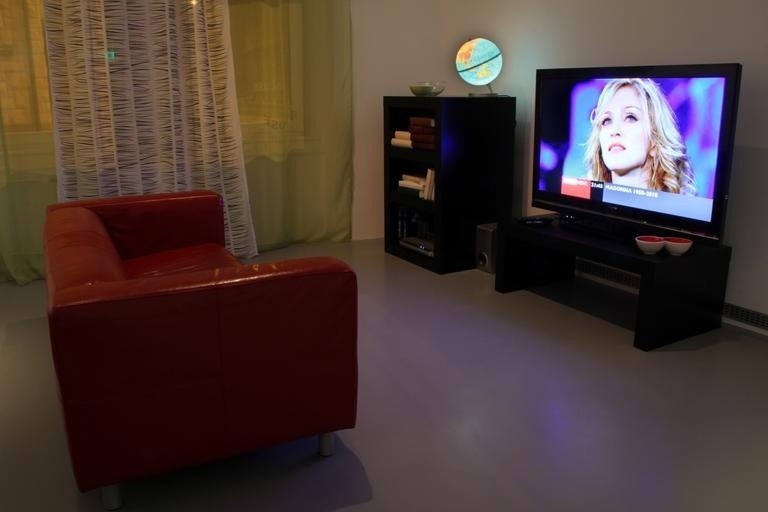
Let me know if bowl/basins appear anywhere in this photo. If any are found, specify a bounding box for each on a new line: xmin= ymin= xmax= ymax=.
xmin=635 ymin=235 xmax=693 ymax=256
xmin=410 ymin=81 xmax=446 ymax=96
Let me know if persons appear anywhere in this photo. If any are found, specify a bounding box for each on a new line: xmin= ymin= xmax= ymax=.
xmin=578 ymin=78 xmax=698 ymax=197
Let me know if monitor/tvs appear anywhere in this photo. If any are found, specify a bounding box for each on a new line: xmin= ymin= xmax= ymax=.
xmin=531 ymin=63 xmax=742 ymax=246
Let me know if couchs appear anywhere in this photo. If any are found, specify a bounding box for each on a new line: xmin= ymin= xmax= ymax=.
xmin=43 ymin=189 xmax=358 ymax=510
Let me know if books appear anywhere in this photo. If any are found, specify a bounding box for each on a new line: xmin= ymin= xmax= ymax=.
xmin=390 ymin=116 xmax=439 ymax=239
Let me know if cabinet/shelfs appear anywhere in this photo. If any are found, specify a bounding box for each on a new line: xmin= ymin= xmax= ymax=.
xmin=493 ymin=211 xmax=734 ymax=352
xmin=383 ymin=96 xmax=516 ymax=276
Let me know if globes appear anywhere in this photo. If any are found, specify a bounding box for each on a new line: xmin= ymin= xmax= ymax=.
xmin=455 ymin=37 xmax=503 ymax=96
